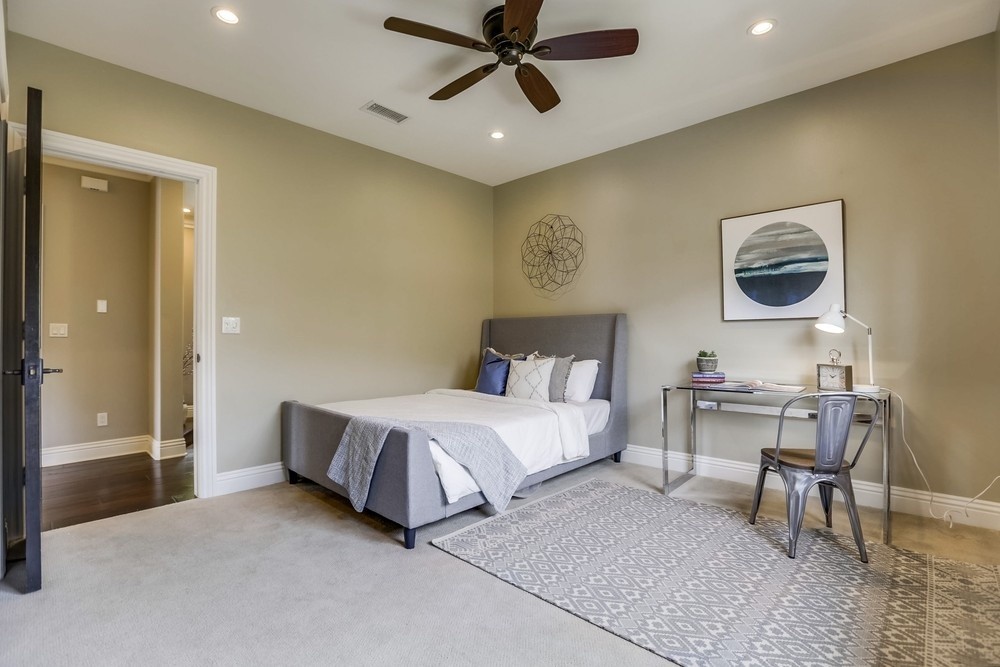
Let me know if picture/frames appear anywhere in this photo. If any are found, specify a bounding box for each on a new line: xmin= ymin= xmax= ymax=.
xmin=719 ymin=199 xmax=846 ymax=321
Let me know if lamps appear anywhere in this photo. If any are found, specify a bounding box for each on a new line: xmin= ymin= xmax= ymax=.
xmin=814 ymin=302 xmax=885 ymax=392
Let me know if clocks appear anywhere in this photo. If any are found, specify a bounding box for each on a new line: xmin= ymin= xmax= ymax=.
xmin=817 ymin=348 xmax=853 ymax=392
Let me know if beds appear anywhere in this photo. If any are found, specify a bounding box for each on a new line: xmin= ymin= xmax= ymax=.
xmin=279 ymin=311 xmax=628 ymax=548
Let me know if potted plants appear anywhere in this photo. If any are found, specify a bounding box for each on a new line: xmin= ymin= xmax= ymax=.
xmin=697 ymin=349 xmax=719 ymax=375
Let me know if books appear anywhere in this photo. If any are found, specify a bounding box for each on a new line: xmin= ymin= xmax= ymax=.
xmin=691 ymin=372 xmax=807 ymax=393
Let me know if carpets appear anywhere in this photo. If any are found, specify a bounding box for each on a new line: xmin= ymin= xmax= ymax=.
xmin=426 ymin=477 xmax=1000 ymax=667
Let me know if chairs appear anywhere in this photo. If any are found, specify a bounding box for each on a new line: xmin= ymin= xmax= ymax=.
xmin=750 ymin=392 xmax=881 ymax=563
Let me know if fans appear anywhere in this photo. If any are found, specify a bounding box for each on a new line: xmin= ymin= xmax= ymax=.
xmin=386 ymin=0 xmax=640 ymax=114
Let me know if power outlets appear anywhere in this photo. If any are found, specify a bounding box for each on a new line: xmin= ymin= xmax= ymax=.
xmin=97 ymin=412 xmax=108 ymax=428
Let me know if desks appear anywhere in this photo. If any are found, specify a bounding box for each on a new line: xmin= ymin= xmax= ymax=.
xmin=661 ymin=381 xmax=894 ymax=546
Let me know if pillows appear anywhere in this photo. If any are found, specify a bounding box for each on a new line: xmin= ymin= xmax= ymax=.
xmin=475 ymin=346 xmax=601 ymax=403
